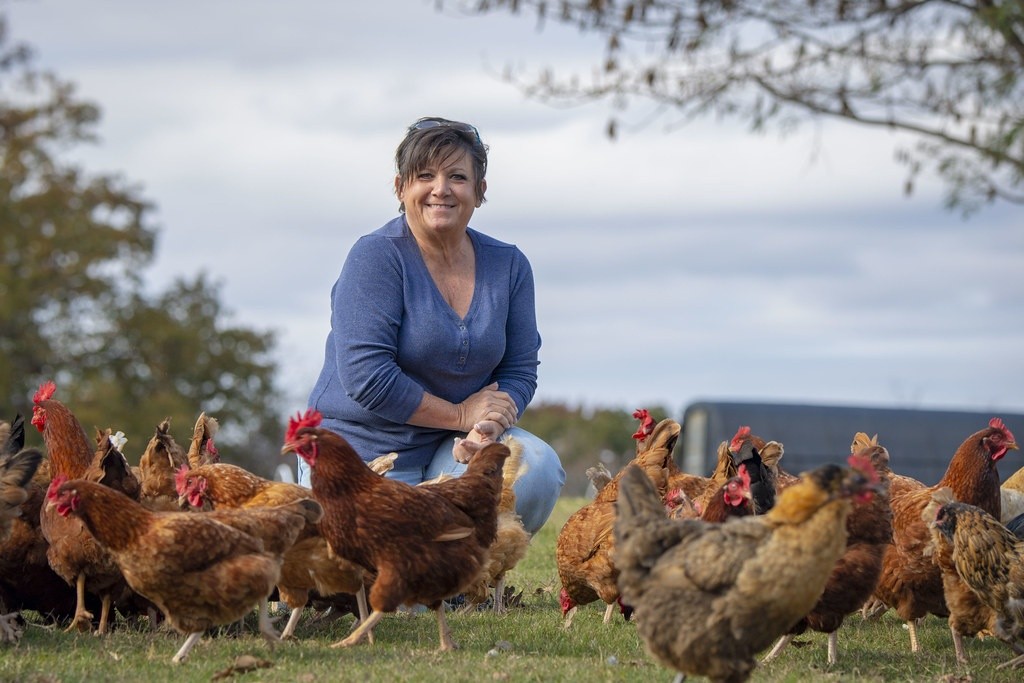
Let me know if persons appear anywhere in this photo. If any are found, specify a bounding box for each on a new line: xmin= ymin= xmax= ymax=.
xmin=272 ymin=117 xmax=566 ymax=611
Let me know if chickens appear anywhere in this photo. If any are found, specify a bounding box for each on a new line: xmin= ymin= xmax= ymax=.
xmin=0 ymin=379 xmax=1024 ymax=683
